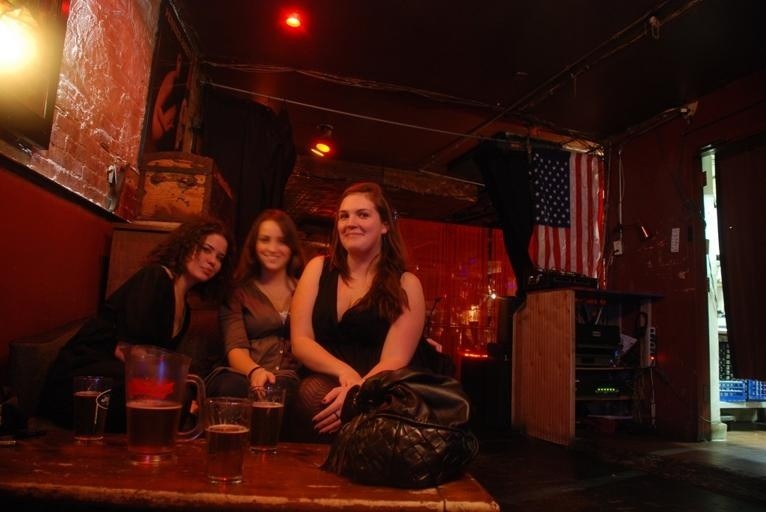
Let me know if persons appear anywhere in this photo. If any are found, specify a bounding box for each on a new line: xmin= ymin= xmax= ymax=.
xmin=208 ymin=206 xmax=307 ymax=441
xmin=289 ymin=180 xmax=428 ymax=437
xmin=38 ymin=213 xmax=237 ymax=422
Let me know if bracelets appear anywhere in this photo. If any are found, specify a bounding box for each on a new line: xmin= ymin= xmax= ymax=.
xmin=248 ymin=367 xmax=261 ymax=379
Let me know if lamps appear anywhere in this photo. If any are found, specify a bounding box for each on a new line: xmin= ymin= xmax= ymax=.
xmin=308 ymin=123 xmax=335 ymax=161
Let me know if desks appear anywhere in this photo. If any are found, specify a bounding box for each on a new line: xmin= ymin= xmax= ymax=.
xmin=103 ymin=220 xmax=182 ymax=301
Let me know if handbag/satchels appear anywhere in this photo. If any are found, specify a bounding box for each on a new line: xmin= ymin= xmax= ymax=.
xmin=320 ymin=368 xmax=480 ymax=490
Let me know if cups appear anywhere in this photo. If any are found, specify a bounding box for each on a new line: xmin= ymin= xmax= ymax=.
xmin=249 ymin=385 xmax=287 ymax=453
xmin=70 ymin=375 xmax=112 ymax=446
xmin=125 ymin=346 xmax=206 ymax=455
xmin=203 ymin=397 xmax=250 ymax=486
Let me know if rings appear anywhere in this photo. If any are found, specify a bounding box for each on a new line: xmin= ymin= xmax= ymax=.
xmin=333 ymin=411 xmax=340 ymax=420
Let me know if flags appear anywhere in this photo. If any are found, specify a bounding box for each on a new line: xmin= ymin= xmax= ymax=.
xmin=526 ymin=149 xmax=608 ymax=290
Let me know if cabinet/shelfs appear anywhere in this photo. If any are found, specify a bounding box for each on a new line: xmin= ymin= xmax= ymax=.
xmin=510 ymin=286 xmax=664 ymax=449
xmin=719 ymin=332 xmax=766 ymax=422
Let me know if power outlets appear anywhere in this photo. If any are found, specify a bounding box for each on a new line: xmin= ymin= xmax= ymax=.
xmin=613 ymin=241 xmax=623 ymax=255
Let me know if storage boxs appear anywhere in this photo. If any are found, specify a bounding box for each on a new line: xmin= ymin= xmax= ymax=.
xmin=135 ymin=151 xmax=237 ymax=231
